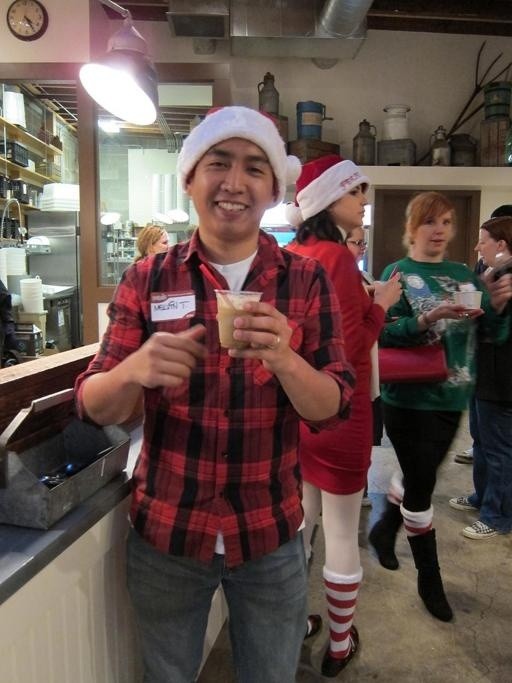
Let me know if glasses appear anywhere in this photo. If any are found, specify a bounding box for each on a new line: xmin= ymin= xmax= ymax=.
xmin=350 ymin=240 xmax=367 ymax=246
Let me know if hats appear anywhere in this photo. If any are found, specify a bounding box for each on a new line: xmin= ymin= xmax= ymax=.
xmin=294 ymin=153 xmax=369 ymax=225
xmin=176 ymin=106 xmax=303 ymax=208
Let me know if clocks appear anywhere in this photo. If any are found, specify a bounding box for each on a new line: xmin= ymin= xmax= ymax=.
xmin=7 ymin=0 xmax=50 ymax=40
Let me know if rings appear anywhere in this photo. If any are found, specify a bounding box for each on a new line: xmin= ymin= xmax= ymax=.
xmin=266 ymin=339 xmax=281 ymax=352
xmin=464 ymin=313 xmax=471 ymax=319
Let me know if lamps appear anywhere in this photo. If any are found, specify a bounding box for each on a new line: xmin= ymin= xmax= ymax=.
xmin=77 ymin=1 xmax=163 ymax=129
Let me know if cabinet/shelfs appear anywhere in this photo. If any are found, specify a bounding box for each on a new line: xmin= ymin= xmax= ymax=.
xmin=1 ymin=117 xmax=64 ymax=242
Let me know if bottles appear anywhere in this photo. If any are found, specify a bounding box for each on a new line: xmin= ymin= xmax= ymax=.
xmin=1 ymin=174 xmax=43 ymax=208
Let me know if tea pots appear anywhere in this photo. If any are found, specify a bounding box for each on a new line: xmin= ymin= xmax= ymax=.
xmin=256 ymin=70 xmax=280 ymax=116
xmin=429 ymin=124 xmax=453 ymax=167
xmin=352 ymin=118 xmax=378 ymax=163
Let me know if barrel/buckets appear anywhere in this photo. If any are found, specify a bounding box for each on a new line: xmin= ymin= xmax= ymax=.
xmin=483 ymin=80 xmax=512 ymax=120
xmin=380 ymin=101 xmax=409 ymax=139
xmin=481 ymin=115 xmax=510 ymax=166
xmin=269 ymin=114 xmax=289 ymax=147
xmin=448 ymin=133 xmax=478 ymax=167
xmin=297 ymin=100 xmax=334 ymax=141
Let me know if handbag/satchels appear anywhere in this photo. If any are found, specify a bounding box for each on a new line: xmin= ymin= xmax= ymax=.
xmin=377 ymin=342 xmax=448 ymax=384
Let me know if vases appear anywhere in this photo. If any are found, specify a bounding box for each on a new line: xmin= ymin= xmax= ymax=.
xmin=381 ymin=102 xmax=413 ymax=139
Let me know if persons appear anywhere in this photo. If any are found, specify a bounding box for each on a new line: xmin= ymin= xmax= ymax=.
xmin=455 ymin=206 xmax=512 ymax=464
xmin=341 ymin=228 xmax=383 ymax=508
xmin=370 ymin=192 xmax=512 ymax=623
xmin=74 ymin=107 xmax=356 ymax=683
xmin=132 ymin=225 xmax=169 ymax=267
xmin=449 ymin=217 xmax=512 ymax=539
xmin=283 ymin=154 xmax=403 ymax=678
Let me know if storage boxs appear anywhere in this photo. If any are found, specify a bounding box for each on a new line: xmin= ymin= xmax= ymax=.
xmin=374 ymin=138 xmax=416 ymax=167
xmin=286 ymin=138 xmax=340 ymax=164
xmin=479 ymin=117 xmax=512 ymax=167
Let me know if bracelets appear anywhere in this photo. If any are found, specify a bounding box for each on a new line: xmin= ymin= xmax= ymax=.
xmin=421 ymin=310 xmax=436 ymax=328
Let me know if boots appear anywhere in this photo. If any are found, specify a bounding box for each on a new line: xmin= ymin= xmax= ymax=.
xmin=368 ymin=502 xmax=403 ymax=569
xmin=407 ymin=527 xmax=453 ymax=621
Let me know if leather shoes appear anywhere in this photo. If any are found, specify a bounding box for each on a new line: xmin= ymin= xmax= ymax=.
xmin=303 ymin=613 xmax=322 ymax=638
xmin=320 ymin=624 xmax=359 ymax=677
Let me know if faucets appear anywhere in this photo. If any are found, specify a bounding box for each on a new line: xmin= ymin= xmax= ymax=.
xmin=0 ymin=197 xmax=24 ymax=244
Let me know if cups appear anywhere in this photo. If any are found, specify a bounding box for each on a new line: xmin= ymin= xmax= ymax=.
xmin=19 ymin=277 xmax=46 ymax=315
xmin=452 ymin=289 xmax=484 ymax=311
xmin=213 ymin=288 xmax=265 ymax=351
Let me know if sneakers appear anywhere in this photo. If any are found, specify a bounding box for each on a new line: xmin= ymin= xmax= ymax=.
xmin=454 ymin=449 xmax=473 ymax=464
xmin=462 ymin=520 xmax=500 ymax=539
xmin=448 ymin=495 xmax=479 ymax=511
